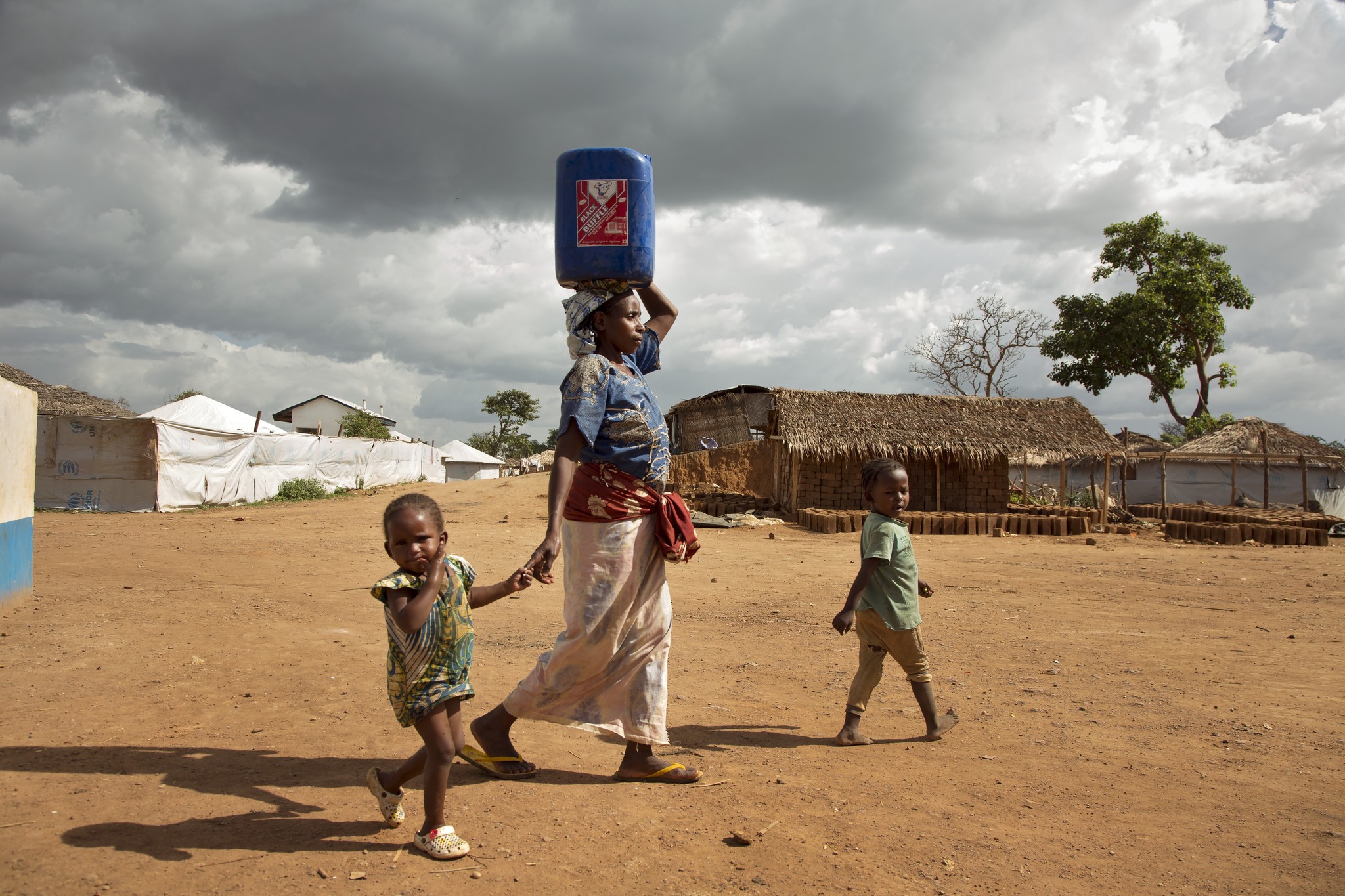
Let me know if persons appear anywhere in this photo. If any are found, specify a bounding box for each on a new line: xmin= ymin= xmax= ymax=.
xmin=832 ymin=458 xmax=959 ymax=745
xmin=366 ymin=494 xmax=534 ymax=861
xmin=460 ymin=281 xmax=703 ymax=782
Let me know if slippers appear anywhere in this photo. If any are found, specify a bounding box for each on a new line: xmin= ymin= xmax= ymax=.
xmin=365 ymin=766 xmax=405 ymax=828
xmin=614 ymin=762 xmax=703 ymax=782
xmin=459 ymin=744 xmax=537 ymax=778
xmin=413 ymin=825 xmax=470 ymax=859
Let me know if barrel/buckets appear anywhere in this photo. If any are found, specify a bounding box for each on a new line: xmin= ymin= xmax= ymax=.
xmin=555 ymin=147 xmax=655 ymax=289
